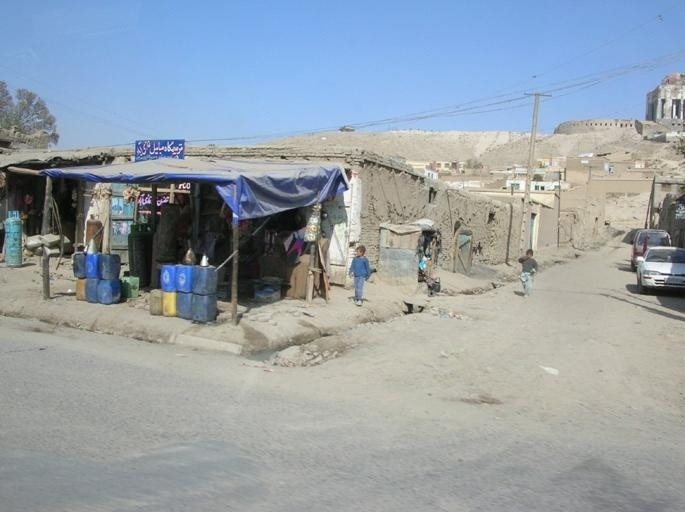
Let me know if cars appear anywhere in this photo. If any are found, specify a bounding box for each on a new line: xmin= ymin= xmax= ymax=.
xmin=634 ymin=246 xmax=685 ymax=298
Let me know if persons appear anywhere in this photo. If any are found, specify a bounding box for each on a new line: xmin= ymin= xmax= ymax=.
xmin=418 ymin=249 xmax=425 ymax=269
xmin=347 ymin=244 xmax=373 ymax=307
xmin=518 ymin=248 xmax=539 ymax=298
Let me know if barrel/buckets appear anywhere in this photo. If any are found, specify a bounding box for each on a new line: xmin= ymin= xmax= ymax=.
xmin=150 ymin=264 xmax=218 ymax=321
xmin=72 ymin=252 xmax=122 ymax=305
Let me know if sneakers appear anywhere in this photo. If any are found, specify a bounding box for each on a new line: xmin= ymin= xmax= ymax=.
xmin=355 ymin=300 xmax=362 ymax=306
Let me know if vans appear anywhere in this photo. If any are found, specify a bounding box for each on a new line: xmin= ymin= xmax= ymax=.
xmin=631 ymin=228 xmax=672 ymax=272
